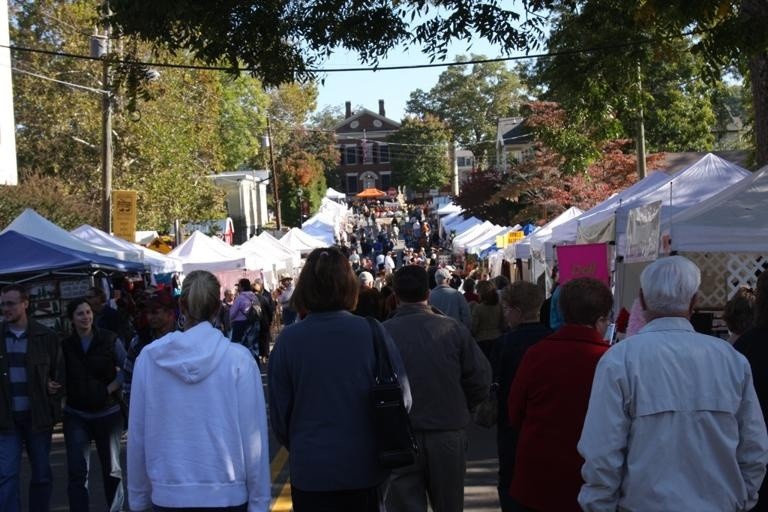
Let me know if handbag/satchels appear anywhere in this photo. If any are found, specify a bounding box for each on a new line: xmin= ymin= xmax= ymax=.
xmin=238 ymin=293 xmax=263 ymax=324
xmin=362 ymin=377 xmax=421 ymax=470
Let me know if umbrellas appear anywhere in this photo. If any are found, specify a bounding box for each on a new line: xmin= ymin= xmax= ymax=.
xmin=356 ymin=188 xmax=387 ymax=198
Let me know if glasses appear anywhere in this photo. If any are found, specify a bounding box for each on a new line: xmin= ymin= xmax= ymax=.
xmin=0 ymin=298 xmax=28 ymax=309
xmin=599 ymin=317 xmax=614 ymax=326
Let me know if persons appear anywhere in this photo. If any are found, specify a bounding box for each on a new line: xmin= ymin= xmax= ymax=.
xmin=0 ymin=199 xmax=768 ymax=512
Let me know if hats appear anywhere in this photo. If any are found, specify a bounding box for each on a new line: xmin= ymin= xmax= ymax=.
xmin=433 ymin=268 xmax=454 ymax=282
xmin=444 ymin=264 xmax=456 ymax=272
xmin=278 ymin=272 xmax=293 ymax=281
xmin=234 ymin=278 xmax=252 ymax=289
xmin=141 ymin=289 xmax=179 ymax=313
